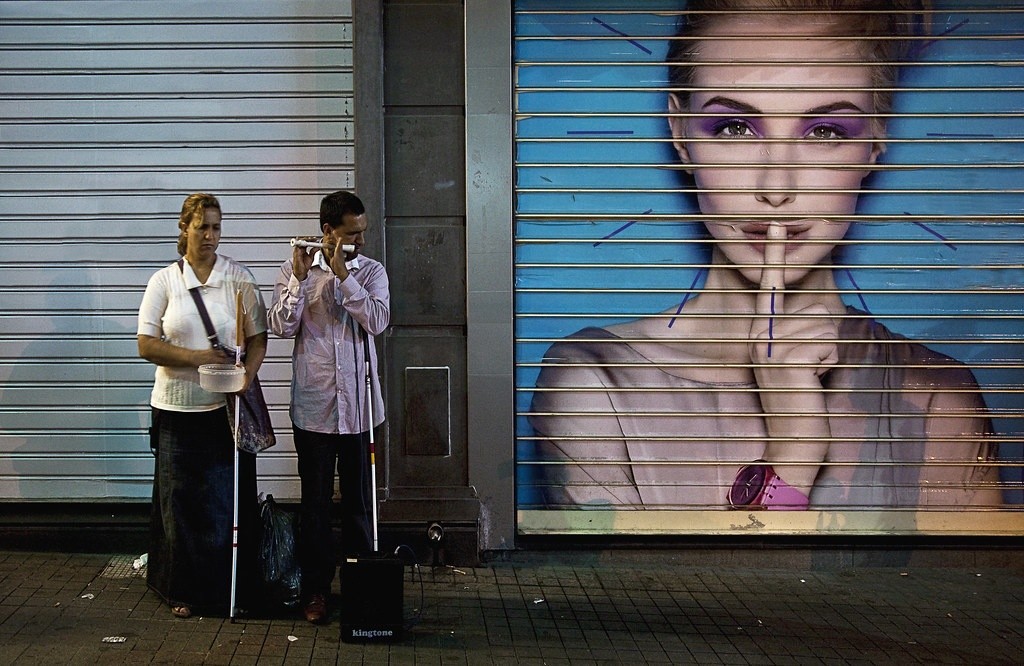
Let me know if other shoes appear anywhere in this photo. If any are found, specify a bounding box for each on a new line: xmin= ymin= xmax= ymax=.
xmin=172 ymin=606 xmax=191 ymax=617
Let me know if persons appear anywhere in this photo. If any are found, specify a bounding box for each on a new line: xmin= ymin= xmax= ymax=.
xmin=530 ymin=0 xmax=1005 ymax=511
xmin=268 ymin=191 xmax=389 ymax=619
xmin=137 ymin=194 xmax=266 ymax=616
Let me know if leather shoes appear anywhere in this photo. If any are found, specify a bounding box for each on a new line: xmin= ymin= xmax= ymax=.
xmin=303 ymin=591 xmax=327 ymax=621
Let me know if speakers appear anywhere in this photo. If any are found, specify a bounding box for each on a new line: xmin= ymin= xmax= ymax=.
xmin=341 ymin=551 xmax=406 ymax=643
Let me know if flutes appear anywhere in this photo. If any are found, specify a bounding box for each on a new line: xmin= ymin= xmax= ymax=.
xmin=291 ymin=237 xmax=355 ymax=254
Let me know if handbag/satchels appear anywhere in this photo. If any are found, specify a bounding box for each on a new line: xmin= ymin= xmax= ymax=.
xmin=256 ymin=494 xmax=300 ymax=619
xmin=215 ymin=346 xmax=276 ymax=453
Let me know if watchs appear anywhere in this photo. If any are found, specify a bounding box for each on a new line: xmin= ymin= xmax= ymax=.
xmin=726 ymin=460 xmax=808 ymax=510
